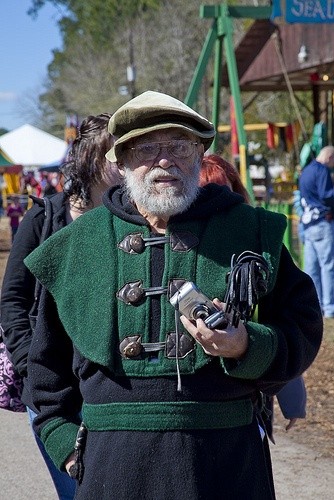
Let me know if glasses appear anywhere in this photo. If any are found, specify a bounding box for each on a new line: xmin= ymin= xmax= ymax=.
xmin=123 ymin=139 xmax=203 ymax=162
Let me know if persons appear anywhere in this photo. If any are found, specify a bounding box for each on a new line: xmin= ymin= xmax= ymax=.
xmin=298 ymin=146 xmax=334 ymax=320
xmin=22 ymin=89 xmax=324 ymax=500
xmin=0 ymin=167 xmax=61 ymax=244
xmin=0 ymin=112 xmax=123 ymax=500
xmin=197 ymin=154 xmax=309 ymax=431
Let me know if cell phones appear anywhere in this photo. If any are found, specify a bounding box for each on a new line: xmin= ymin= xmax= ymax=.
xmin=204 ymin=311 xmax=227 ymax=330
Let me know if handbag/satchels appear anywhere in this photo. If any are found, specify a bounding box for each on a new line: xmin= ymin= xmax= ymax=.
xmin=0 ymin=194 xmax=50 ymax=413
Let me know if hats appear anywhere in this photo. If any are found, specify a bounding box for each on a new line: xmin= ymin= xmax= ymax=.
xmin=105 ymin=90 xmax=217 ymax=163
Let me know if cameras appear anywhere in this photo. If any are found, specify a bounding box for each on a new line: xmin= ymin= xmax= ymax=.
xmin=170 ymin=282 xmax=220 ymax=326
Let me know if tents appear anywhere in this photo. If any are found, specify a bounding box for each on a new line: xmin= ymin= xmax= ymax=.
xmin=0 ymin=123 xmax=69 ymax=168
